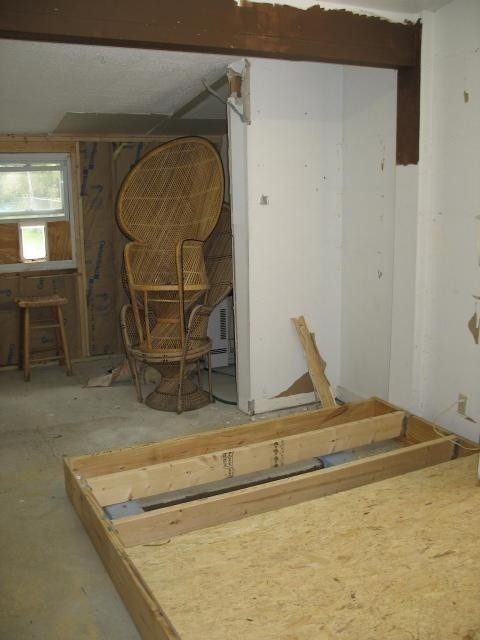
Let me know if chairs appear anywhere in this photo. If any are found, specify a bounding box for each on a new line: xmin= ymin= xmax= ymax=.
xmin=115 ymin=136 xmax=233 ymax=414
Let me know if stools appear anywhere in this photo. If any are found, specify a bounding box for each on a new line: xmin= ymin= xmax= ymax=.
xmin=14 ymin=294 xmax=73 ymax=382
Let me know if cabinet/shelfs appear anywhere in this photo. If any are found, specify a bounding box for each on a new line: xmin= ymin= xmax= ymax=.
xmin=62 ymin=397 xmax=480 ymax=640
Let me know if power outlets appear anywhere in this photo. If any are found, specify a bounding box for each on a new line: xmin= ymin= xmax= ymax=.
xmin=456 ymin=393 xmax=469 ymax=416
